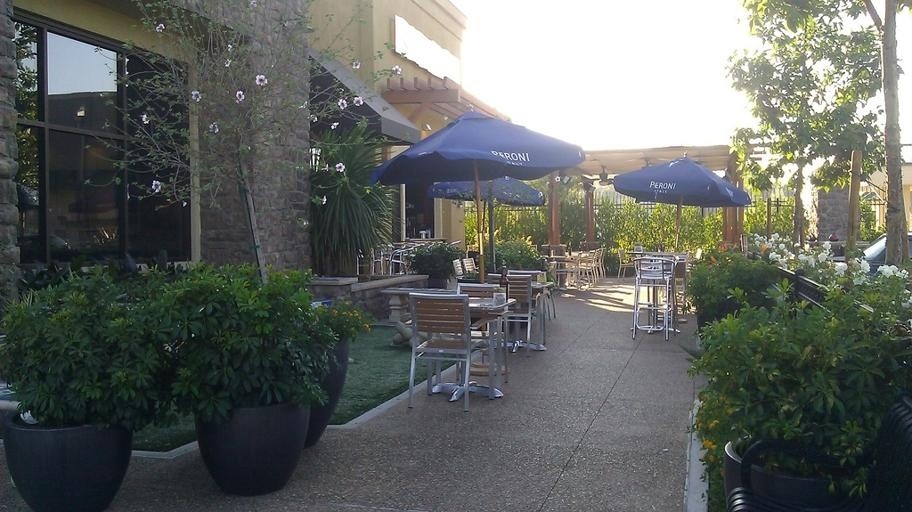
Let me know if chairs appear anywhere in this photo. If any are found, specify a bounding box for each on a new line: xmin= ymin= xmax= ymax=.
xmin=380 ymin=270 xmax=556 ymax=411
xmin=533 ymin=236 xmax=713 ymax=341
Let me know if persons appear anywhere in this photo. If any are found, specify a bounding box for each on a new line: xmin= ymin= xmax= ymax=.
xmin=806 ymin=231 xmax=839 ymax=250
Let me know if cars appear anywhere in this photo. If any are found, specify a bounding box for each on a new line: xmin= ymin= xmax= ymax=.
xmin=827 ymin=228 xmax=912 ymax=276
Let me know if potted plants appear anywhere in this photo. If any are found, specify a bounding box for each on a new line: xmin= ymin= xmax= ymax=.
xmin=682 ymin=252 xmax=780 ymax=352
xmin=313 ymin=121 xmax=409 ymax=281
xmin=2 ymin=260 xmax=177 ymax=505
xmin=164 ymin=258 xmax=378 ymax=497
xmin=683 ymin=274 xmax=912 ymax=512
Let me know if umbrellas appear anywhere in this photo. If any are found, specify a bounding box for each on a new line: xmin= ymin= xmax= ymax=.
xmin=423 ymin=172 xmax=547 ymax=281
xmin=366 ymin=111 xmax=586 ymax=342
xmin=612 ymin=150 xmax=754 ymax=258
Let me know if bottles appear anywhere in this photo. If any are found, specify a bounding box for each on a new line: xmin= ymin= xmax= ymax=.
xmin=500 ymin=260 xmax=509 ymax=301
